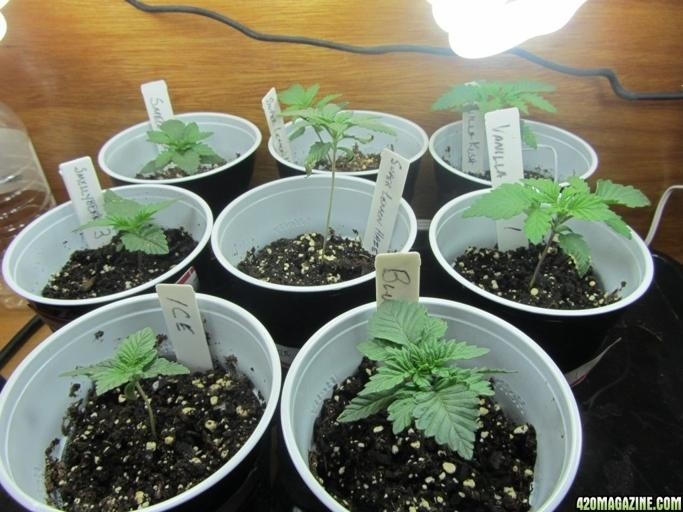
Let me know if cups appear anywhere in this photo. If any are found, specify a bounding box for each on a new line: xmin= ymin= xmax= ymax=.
xmin=0 ymin=111 xmax=654 ymax=512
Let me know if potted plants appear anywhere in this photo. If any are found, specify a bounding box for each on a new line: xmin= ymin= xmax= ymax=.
xmin=1 ymin=73 xmax=656 ymax=512
